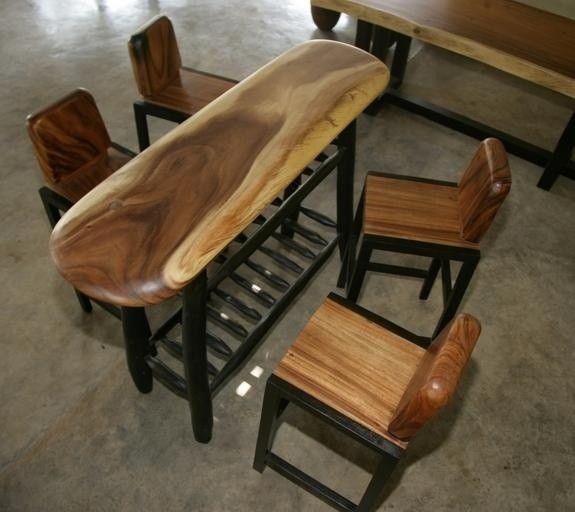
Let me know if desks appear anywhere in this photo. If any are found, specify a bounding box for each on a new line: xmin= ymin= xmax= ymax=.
xmin=47 ymin=41 xmax=389 ymax=441
xmin=311 ymin=0 xmax=575 ymax=192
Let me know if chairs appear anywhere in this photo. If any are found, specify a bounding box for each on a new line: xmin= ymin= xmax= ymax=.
xmin=338 ymin=135 xmax=512 ymax=341
xmin=126 ymin=14 xmax=238 ymax=152
xmin=25 ymin=87 xmax=138 ymax=230
xmin=253 ymin=293 xmax=482 ymax=512
